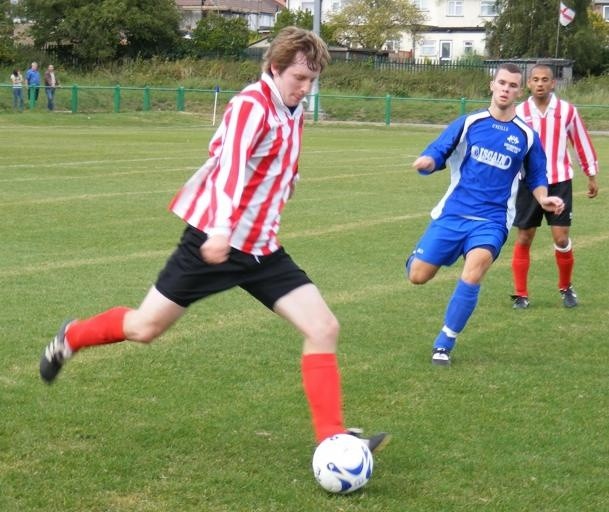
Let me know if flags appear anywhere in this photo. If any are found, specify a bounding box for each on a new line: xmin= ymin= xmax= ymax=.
xmin=559 ymin=1 xmax=576 ymax=27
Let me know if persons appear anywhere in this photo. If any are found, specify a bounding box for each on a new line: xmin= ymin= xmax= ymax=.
xmin=44 ymin=63 xmax=61 ymax=111
xmin=9 ymin=68 xmax=25 ymax=113
xmin=406 ymin=59 xmax=567 ymax=367
xmin=36 ymin=22 xmax=390 ymax=467
xmin=508 ymin=63 xmax=601 ymax=310
xmin=24 ymin=60 xmax=42 ymax=109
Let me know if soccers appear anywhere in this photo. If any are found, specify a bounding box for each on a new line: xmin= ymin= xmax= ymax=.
xmin=312 ymin=434 xmax=373 ymax=494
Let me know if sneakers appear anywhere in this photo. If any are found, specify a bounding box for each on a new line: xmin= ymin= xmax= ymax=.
xmin=558 ymin=281 xmax=577 ymax=309
xmin=39 ymin=316 xmax=80 ymax=384
xmin=429 ymin=347 xmax=452 ymax=366
xmin=348 ymin=425 xmax=392 ymax=452
xmin=509 ymin=294 xmax=529 ymax=309
